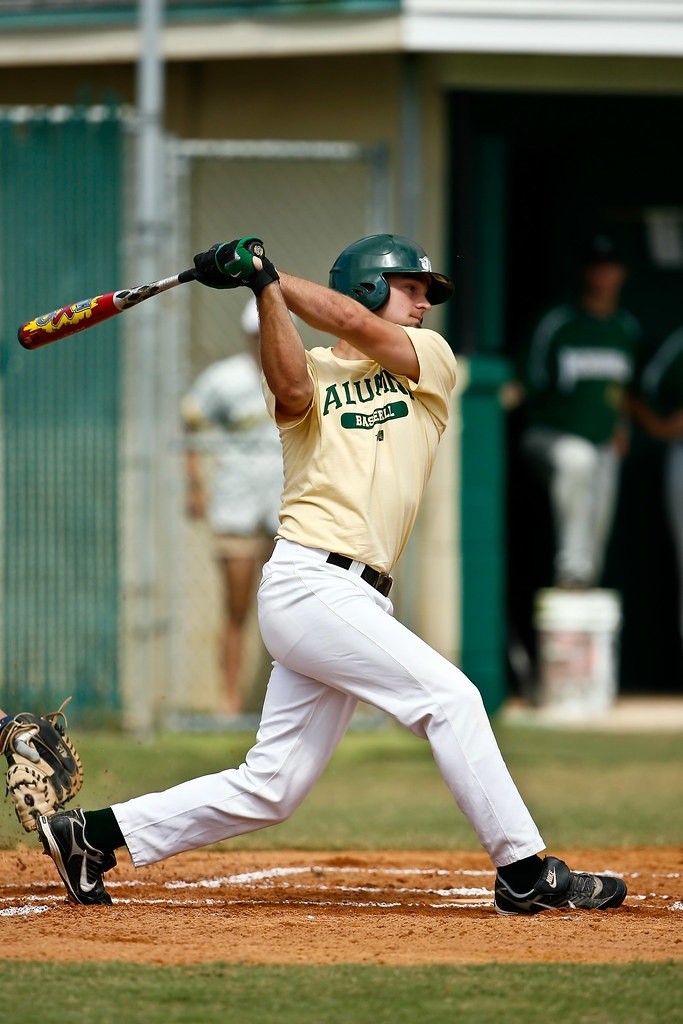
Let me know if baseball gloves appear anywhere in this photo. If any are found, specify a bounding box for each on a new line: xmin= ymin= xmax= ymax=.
xmin=0 ymin=712 xmax=84 ymax=834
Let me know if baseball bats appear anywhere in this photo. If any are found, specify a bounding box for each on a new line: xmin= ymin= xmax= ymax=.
xmin=17 ymin=241 xmax=264 ymax=351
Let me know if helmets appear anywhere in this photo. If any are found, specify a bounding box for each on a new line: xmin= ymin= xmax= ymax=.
xmin=328 ymin=233 xmax=452 ymax=312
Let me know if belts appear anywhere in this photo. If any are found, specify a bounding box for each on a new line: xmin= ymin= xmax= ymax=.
xmin=326 ymin=551 xmax=392 ymax=598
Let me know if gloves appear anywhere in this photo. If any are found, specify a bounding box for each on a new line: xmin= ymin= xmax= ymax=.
xmin=192 ymin=236 xmax=279 ymax=293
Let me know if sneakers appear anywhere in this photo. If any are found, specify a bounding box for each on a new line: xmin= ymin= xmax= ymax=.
xmin=36 ymin=806 xmax=118 ymax=906
xmin=495 ymin=856 xmax=627 ymax=917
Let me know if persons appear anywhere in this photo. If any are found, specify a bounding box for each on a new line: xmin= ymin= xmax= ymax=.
xmin=0 ymin=708 xmax=84 ymax=832
xmin=179 ymin=294 xmax=297 ymax=722
xmin=38 ymin=234 xmax=628 ymax=914
xmin=526 ymin=238 xmax=683 ymax=586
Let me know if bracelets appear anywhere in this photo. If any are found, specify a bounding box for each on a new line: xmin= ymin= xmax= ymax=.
xmin=0 ymin=716 xmax=14 ymax=732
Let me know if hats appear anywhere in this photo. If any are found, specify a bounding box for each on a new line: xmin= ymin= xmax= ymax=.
xmin=580 ymin=237 xmax=624 ymax=267
xmin=242 ymin=295 xmax=297 ymax=330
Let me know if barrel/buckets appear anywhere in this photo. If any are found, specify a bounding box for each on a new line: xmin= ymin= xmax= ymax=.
xmin=537 ymin=587 xmax=620 ymax=716
